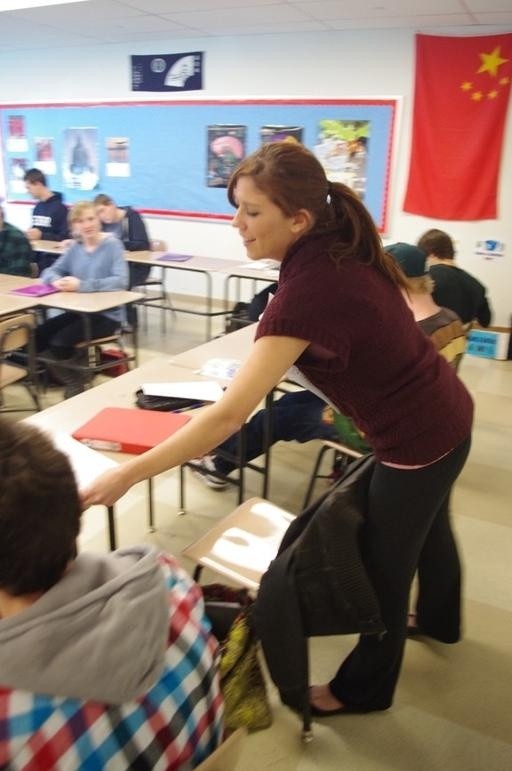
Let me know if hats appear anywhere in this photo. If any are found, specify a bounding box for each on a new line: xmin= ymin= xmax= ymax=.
xmin=384 ymin=242 xmax=427 ymax=277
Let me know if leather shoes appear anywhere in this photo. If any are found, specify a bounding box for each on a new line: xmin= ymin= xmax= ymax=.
xmin=307 ymin=685 xmax=359 ymax=716
xmin=407 ymin=614 xmax=420 ymax=636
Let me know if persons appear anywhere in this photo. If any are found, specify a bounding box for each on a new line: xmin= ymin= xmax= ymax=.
xmin=93 ymin=193 xmax=153 ymax=335
xmin=0 ymin=206 xmax=34 ymax=279
xmin=178 ymin=241 xmax=464 ymax=490
xmin=37 ymin=199 xmax=132 ymax=400
xmin=72 ymin=140 xmax=478 ymax=720
xmin=417 ymin=229 xmax=492 ymax=331
xmin=23 ymin=169 xmax=70 ymax=275
xmin=0 ymin=415 xmax=229 ymax=771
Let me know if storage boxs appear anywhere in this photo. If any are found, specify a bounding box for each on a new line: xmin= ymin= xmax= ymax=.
xmin=464 ymin=321 xmax=511 ymax=360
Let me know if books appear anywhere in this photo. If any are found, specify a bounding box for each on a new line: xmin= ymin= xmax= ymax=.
xmin=70 ymin=406 xmax=192 ymax=454
xmin=8 ymin=283 xmax=60 ymax=297
xmin=156 ymin=251 xmax=193 ymax=262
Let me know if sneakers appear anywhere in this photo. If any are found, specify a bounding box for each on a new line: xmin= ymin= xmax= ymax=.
xmin=186 ymin=453 xmax=230 ymax=489
xmin=64 ymin=379 xmax=84 ymax=398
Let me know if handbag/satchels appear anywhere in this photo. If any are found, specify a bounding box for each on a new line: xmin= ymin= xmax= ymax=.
xmin=201 ymin=583 xmax=253 ymax=640
xmin=102 ymin=350 xmax=129 ymax=377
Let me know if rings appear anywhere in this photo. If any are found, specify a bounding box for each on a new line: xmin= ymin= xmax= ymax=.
xmin=62 ymin=286 xmax=67 ymax=291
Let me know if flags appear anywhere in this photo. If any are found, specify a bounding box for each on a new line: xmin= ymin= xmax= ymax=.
xmin=400 ymin=30 xmax=512 ymax=221
